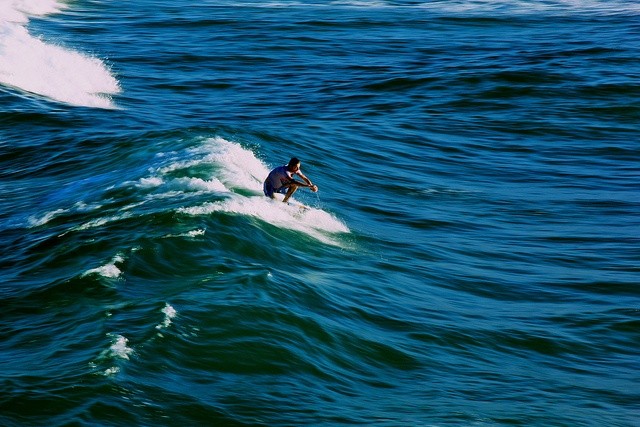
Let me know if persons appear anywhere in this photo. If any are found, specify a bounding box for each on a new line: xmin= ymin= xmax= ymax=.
xmin=263 ymin=158 xmax=318 ymax=205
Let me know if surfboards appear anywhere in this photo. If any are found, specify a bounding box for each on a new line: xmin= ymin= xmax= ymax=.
xmin=287 ymin=202 xmax=310 ymax=212
